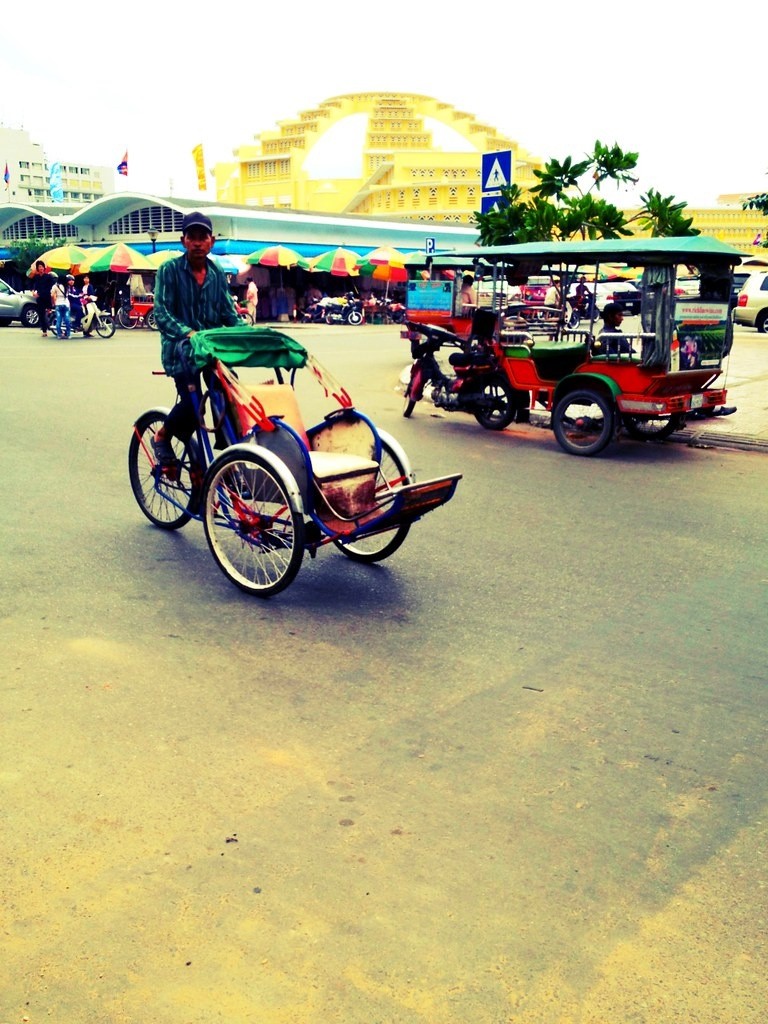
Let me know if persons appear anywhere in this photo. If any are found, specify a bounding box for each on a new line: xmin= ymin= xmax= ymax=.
xmin=51 ymin=274 xmax=71 ymax=339
xmin=461 ymin=275 xmax=479 ymax=303
xmin=81 ymin=275 xmax=94 ymax=295
xmin=31 ymin=261 xmax=55 ymax=336
xmin=151 ymin=213 xmax=247 ymax=465
xmin=598 ymin=303 xmax=636 ymax=355
xmin=577 ymin=276 xmax=593 ymax=319
xmin=544 ymin=280 xmax=560 ymax=308
xmin=245 ymin=277 xmax=258 ymax=322
xmin=305 ymin=285 xmax=322 ymax=304
xmin=66 ymin=274 xmax=94 ymax=336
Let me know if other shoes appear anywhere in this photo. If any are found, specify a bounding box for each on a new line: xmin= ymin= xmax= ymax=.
xmin=43 ymin=332 xmax=48 ymax=336
xmin=84 ymin=331 xmax=94 ymax=337
xmin=58 ymin=336 xmax=61 ymax=339
xmin=66 ymin=335 xmax=71 ymax=339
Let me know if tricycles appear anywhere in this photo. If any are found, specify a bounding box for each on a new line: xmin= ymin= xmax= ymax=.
xmin=128 ymin=324 xmax=461 ymax=596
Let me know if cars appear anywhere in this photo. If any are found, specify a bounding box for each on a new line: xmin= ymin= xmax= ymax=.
xmin=675 ymin=276 xmax=700 ymax=294
xmin=0 ymin=278 xmax=40 ymax=327
xmin=602 ymin=282 xmax=642 ymax=315
xmin=545 ymin=283 xmax=613 ymax=311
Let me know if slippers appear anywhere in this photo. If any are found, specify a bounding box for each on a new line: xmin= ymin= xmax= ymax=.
xmin=150 ymin=435 xmax=177 ymax=466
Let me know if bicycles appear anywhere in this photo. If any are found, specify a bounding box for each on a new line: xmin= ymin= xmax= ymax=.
xmin=106 ymin=290 xmax=138 ymax=329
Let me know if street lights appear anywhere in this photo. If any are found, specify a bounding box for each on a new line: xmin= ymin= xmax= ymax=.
xmin=148 ymin=226 xmax=158 ymax=254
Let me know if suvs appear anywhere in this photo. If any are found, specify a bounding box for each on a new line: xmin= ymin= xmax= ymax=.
xmin=732 ymin=273 xmax=751 ymax=299
xmin=733 ymin=271 xmax=768 ymax=333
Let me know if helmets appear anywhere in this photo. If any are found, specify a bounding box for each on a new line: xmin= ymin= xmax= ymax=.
xmin=67 ymin=274 xmax=75 ymax=281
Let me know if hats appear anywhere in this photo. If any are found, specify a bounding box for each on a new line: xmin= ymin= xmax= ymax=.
xmin=182 ymin=212 xmax=212 ymax=236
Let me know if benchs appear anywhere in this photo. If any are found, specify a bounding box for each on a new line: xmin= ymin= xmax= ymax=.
xmin=584 ymin=346 xmax=647 ymax=373
xmin=518 ymin=329 xmax=594 ymax=370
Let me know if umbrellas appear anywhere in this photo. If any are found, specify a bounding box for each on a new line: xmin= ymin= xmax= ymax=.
xmin=242 ymin=245 xmax=426 ymax=299
xmin=570 ymin=264 xmax=644 ymax=281
xmin=26 ymin=242 xmax=185 ymax=273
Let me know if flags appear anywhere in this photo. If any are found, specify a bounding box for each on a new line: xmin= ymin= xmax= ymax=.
xmin=49 ymin=162 xmax=63 ymax=203
xmin=118 ymin=152 xmax=127 ymax=175
xmin=192 ymin=145 xmax=206 ymax=191
xmin=4 ymin=164 xmax=10 ymax=184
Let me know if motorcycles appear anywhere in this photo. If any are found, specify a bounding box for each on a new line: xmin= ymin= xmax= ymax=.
xmin=49 ymin=296 xmax=115 ymax=339
xmin=559 ymin=299 xmax=580 ymax=329
xmin=400 ymin=320 xmax=530 ymax=430
xmin=232 ymin=296 xmax=254 ymax=326
xmin=325 ymin=299 xmax=363 ymax=326
xmin=570 ymin=293 xmax=600 ymax=323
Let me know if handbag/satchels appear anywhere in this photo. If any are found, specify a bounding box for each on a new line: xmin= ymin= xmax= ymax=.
xmin=462 ymin=292 xmax=475 ymax=315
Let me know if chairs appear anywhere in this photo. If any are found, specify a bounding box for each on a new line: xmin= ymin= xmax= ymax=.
xmin=237 ymin=385 xmax=389 ymax=513
